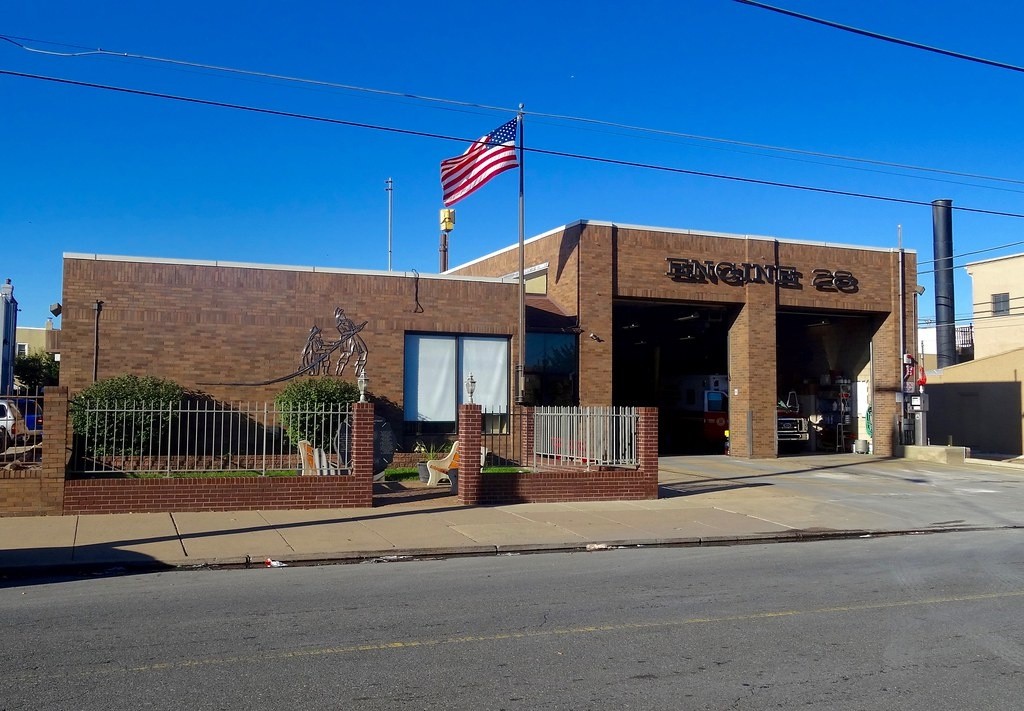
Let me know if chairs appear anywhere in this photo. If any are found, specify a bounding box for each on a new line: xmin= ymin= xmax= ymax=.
xmin=298 ymin=440 xmax=337 ymax=477
xmin=427 ymin=440 xmax=488 ymax=493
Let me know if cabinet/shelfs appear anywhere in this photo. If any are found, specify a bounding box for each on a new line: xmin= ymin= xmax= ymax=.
xmin=816 ymin=386 xmax=851 ymax=451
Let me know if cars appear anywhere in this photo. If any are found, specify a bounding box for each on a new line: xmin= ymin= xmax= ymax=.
xmin=0 ymin=398 xmax=43 ymax=449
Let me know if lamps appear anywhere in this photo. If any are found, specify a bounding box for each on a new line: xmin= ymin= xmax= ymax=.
xmin=915 ymin=285 xmax=925 ymax=295
xmin=50 ymin=303 xmax=61 ymax=317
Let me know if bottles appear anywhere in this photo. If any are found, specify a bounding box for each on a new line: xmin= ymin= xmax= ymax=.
xmin=833 ymin=401 xmax=837 ymax=411
xmin=820 ymin=375 xmax=851 ymax=384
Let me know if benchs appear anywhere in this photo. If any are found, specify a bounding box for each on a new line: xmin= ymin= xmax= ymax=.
xmin=551 ymin=436 xmax=606 ymax=463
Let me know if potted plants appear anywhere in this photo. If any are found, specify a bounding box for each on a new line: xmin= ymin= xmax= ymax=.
xmin=416 ymin=439 xmax=447 ymax=482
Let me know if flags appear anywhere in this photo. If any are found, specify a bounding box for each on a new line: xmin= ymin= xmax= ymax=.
xmin=440 ymin=115 xmax=524 ymax=208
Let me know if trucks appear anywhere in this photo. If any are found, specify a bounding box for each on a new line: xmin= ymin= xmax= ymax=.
xmin=673 ymin=368 xmax=809 ymax=443
